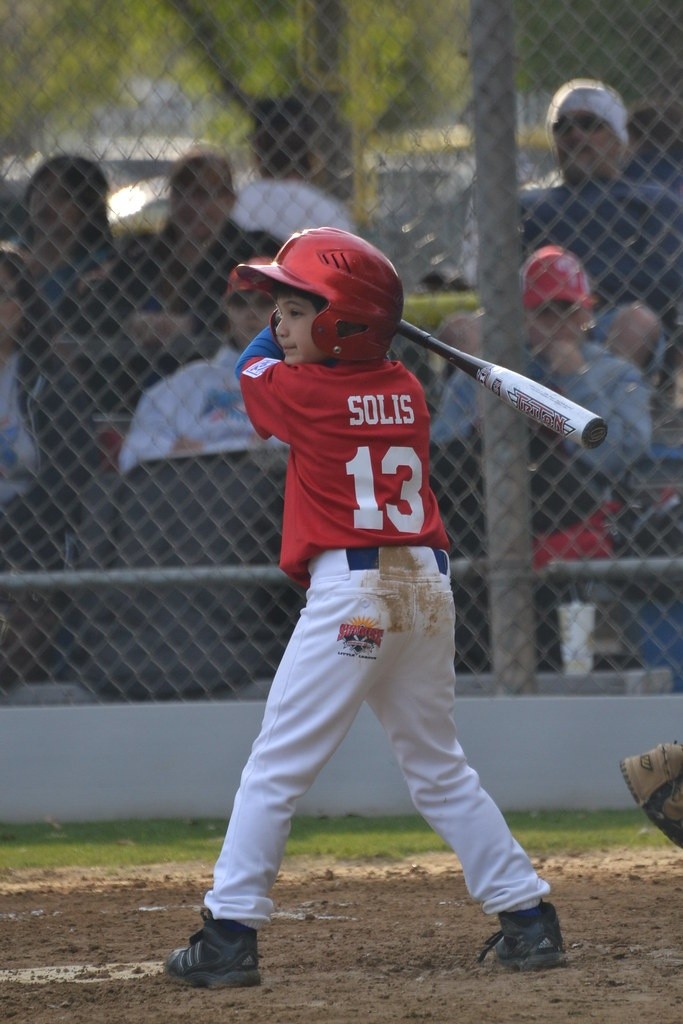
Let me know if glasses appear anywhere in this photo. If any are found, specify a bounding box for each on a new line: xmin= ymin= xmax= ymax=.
xmin=551 ymin=114 xmax=605 ymax=135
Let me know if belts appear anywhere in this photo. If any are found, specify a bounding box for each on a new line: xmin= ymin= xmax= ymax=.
xmin=345 ymin=545 xmax=448 ymax=575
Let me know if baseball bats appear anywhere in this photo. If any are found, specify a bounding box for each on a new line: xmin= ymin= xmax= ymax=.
xmin=400 ymin=319 xmax=611 ymax=453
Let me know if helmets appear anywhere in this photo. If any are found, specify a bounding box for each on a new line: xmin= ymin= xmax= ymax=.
xmin=234 ymin=226 xmax=404 ymax=362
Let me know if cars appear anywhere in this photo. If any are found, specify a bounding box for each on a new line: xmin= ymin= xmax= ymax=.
xmin=2 ymin=104 xmax=569 ymax=242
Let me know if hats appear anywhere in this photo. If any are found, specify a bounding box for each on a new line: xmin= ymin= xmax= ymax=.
xmin=544 ymin=78 xmax=630 ymax=156
xmin=226 ymin=256 xmax=278 ymax=302
xmin=520 ymin=245 xmax=598 ymax=309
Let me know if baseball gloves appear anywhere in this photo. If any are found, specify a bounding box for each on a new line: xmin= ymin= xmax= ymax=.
xmin=618 ymin=741 xmax=683 ymax=852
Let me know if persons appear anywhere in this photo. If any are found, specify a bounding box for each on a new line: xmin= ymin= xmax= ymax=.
xmin=162 ymin=228 xmax=566 ymax=987
xmin=1 ymin=77 xmax=683 ymax=503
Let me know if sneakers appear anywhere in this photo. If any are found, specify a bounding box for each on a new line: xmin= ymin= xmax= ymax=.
xmin=478 ymin=902 xmax=567 ymax=971
xmin=164 ymin=908 xmax=262 ymax=989
